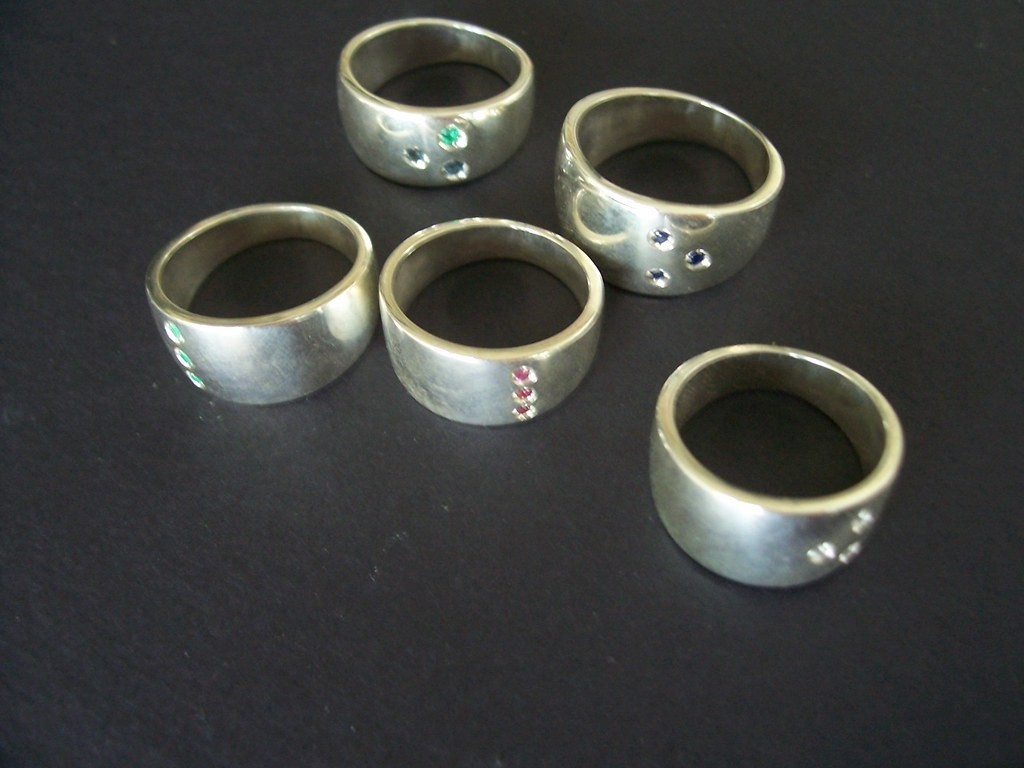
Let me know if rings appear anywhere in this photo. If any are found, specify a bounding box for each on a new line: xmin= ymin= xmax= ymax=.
xmin=380 ymin=218 xmax=604 ymax=425
xmin=144 ymin=203 xmax=380 ymax=406
xmin=337 ymin=18 xmax=535 ymax=188
xmin=555 ymin=89 xmax=786 ymax=296
xmin=652 ymin=344 xmax=903 ymax=589
